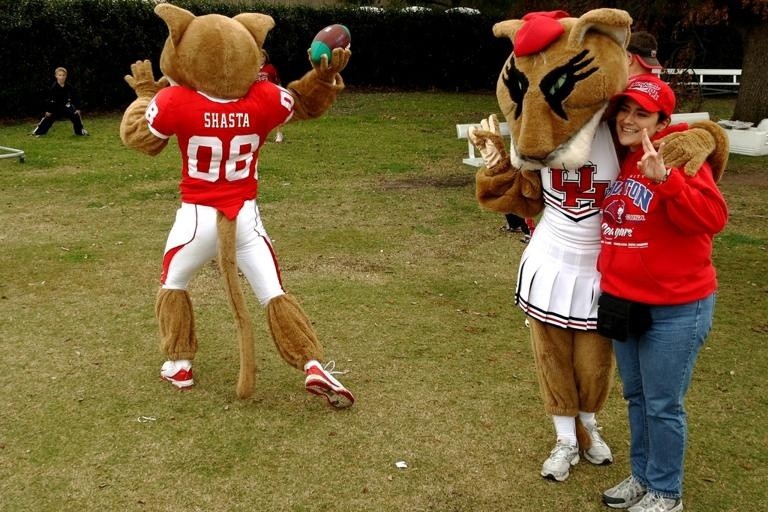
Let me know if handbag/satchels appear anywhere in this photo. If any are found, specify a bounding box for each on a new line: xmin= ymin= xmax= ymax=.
xmin=597 ymin=293 xmax=653 ymax=343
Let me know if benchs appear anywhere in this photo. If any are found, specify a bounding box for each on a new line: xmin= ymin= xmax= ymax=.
xmin=455 ymin=112 xmax=711 ymax=168
xmin=723 ymin=119 xmax=768 ymax=156
xmin=652 ymin=69 xmax=742 ymax=95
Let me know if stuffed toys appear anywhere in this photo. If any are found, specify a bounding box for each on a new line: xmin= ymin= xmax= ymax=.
xmin=467 ymin=7 xmax=728 ymax=483
xmin=119 ymin=2 xmax=355 ymax=412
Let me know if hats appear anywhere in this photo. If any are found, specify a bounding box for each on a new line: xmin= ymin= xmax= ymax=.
xmin=614 ymin=75 xmax=676 ymax=115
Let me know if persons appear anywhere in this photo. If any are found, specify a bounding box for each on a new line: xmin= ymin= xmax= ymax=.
xmin=627 ymin=31 xmax=661 ymax=74
xmin=257 ymin=49 xmax=284 ymax=143
xmin=596 ymin=74 xmax=730 ymax=512
xmin=27 ymin=67 xmax=89 ymax=137
xmin=499 ymin=213 xmax=536 ymax=244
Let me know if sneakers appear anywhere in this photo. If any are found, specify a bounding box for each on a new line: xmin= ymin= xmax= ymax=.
xmin=602 ymin=476 xmax=648 ymax=508
xmin=627 ymin=492 xmax=683 ymax=512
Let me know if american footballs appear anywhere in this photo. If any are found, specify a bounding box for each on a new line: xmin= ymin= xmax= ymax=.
xmin=310 ymin=24 xmax=351 ymax=64
